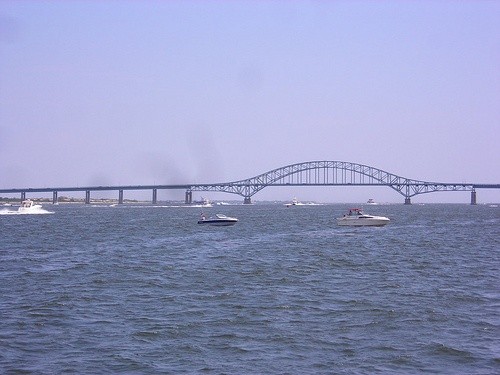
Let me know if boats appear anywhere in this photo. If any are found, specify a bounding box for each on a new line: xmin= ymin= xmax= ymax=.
xmin=281 ymin=197 xmax=325 ymax=207
xmin=364 ymin=198 xmax=379 ymax=205
xmin=4 ymin=200 xmax=42 ymax=208
xmin=192 ymin=197 xmax=216 ymax=206
xmin=8 ymin=203 xmax=44 ymax=214
xmin=335 ymin=208 xmax=391 ymax=227
xmin=198 ymin=211 xmax=237 ymax=226
